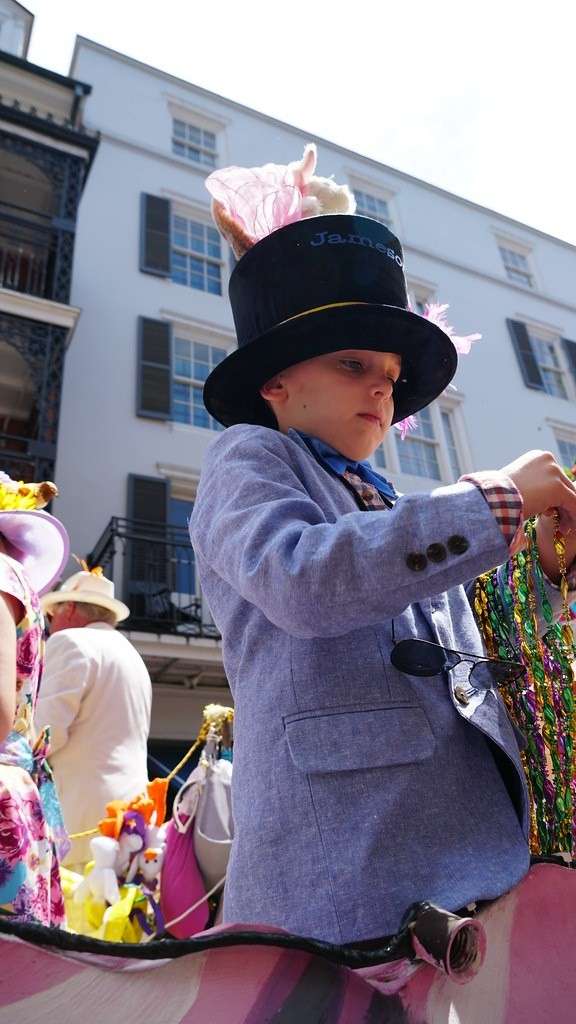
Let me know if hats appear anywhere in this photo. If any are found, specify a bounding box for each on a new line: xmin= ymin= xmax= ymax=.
xmin=38 ymin=571 xmax=130 ymax=622
xmin=202 ymin=213 xmax=459 ymax=437
xmin=0 ymin=508 xmax=70 ymax=597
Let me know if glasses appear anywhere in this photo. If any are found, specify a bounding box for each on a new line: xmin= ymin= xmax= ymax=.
xmin=390 ymin=576 xmax=527 ymax=691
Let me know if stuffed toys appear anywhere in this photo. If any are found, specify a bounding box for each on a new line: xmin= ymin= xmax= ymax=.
xmin=69 ymin=778 xmax=170 ymax=945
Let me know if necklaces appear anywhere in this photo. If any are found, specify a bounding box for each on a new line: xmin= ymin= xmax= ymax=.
xmin=473 ymin=486 xmax=575 ymax=866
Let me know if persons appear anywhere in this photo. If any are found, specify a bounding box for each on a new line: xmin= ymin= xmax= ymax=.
xmin=187 ymin=216 xmax=576 ymax=948
xmin=31 ymin=570 xmax=153 ymax=874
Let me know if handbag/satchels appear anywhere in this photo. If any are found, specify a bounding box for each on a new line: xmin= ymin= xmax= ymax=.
xmin=182 ymin=727 xmax=241 ymax=892
